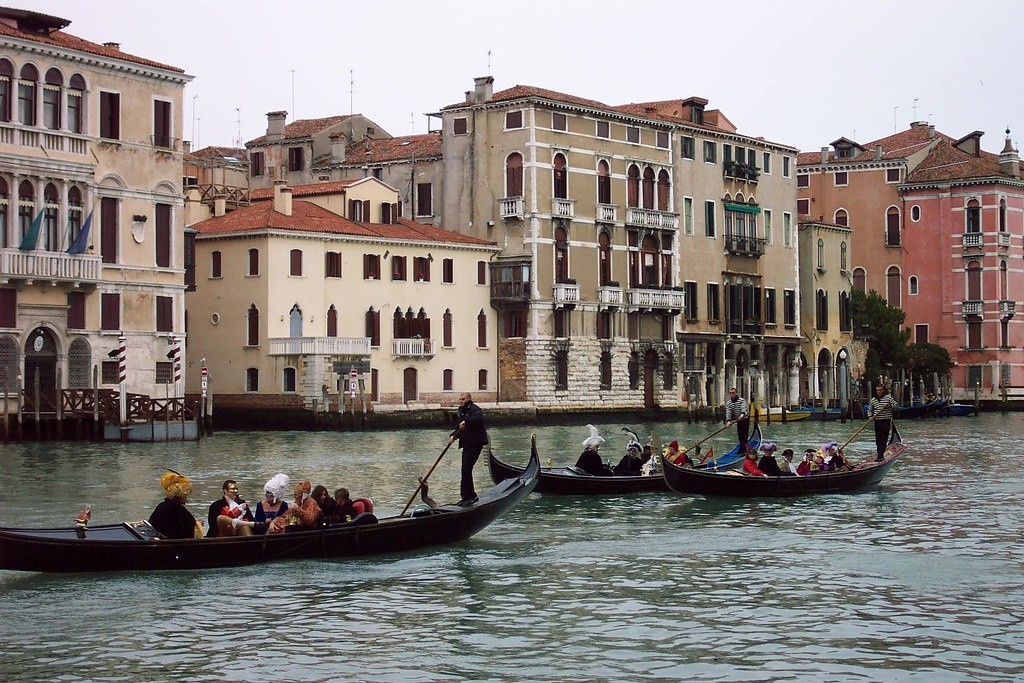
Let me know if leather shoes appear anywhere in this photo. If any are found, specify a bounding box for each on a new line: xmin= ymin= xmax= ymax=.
xmin=874 ymin=457 xmax=884 ymax=462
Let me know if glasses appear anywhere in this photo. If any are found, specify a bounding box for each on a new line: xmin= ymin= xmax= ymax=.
xmin=729 ymin=392 xmax=735 ymax=394
xmin=806 ymin=454 xmax=813 ymax=457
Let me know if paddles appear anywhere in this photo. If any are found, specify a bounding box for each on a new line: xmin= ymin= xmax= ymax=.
xmin=399 ymin=426 xmax=462 ymax=515
xmin=683 ymin=418 xmax=739 ymax=455
xmin=838 ymin=405 xmax=888 ymax=451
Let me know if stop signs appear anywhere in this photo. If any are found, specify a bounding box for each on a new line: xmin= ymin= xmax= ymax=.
xmin=201 ymin=367 xmax=207 ymax=376
xmin=351 ymin=369 xmax=357 ymax=378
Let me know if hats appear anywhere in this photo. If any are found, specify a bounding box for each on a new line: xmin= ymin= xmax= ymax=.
xmin=627 ymin=441 xmax=643 ymax=453
xmin=263 ymin=474 xmax=289 ymax=504
xmin=669 ymin=441 xmax=678 ymax=452
xmin=803 ymin=449 xmax=816 ymax=453
xmin=582 ymin=436 xmax=605 ymax=446
xmin=161 ymin=473 xmax=191 ymax=495
xmin=782 ymin=449 xmax=794 ymax=455
xmin=816 ymin=450 xmax=824 ymax=459
xmin=759 ymin=443 xmax=777 ymax=452
xmin=744 ymin=443 xmax=757 ymax=453
xmin=295 ymin=481 xmax=311 ymax=494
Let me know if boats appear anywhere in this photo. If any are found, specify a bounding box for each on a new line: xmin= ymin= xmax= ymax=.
xmin=480 ymin=405 xmax=762 ymax=495
xmin=653 ymin=416 xmax=907 ymax=498
xmin=748 ymin=395 xmax=978 ymax=422
xmin=0 ymin=433 xmax=546 ymax=572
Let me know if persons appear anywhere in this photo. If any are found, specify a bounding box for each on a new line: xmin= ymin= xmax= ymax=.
xmin=575 ymin=424 xmax=614 ymax=476
xmin=867 ymin=383 xmax=898 ymax=462
xmin=450 ymin=392 xmax=486 ymax=507
xmin=756 ymin=442 xmax=785 ymax=477
xmin=147 ymin=470 xmax=204 ymax=540
xmin=255 ymin=473 xmax=357 ymax=538
xmin=742 ymin=449 xmax=769 ymax=480
xmin=796 ymin=442 xmax=853 ymax=476
xmin=924 ymin=392 xmax=951 ymax=405
xmin=725 ymin=388 xmax=750 ymax=454
xmin=206 ymin=480 xmax=264 ymax=536
xmin=778 ymin=449 xmax=802 ymax=475
xmin=611 ymin=428 xmax=644 ymax=476
xmin=641 ymin=441 xmax=685 ymax=475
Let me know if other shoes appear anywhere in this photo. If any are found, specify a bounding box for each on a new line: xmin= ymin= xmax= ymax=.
xmin=735 ymin=450 xmax=745 ymax=457
xmin=254 ymin=522 xmax=264 ymax=528
xmin=457 ymin=496 xmax=478 ymax=506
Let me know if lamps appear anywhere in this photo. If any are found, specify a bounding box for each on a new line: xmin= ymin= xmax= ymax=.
xmin=108 ymin=349 xmax=121 ymax=359
xmin=166 ymin=350 xmax=175 ymax=361
